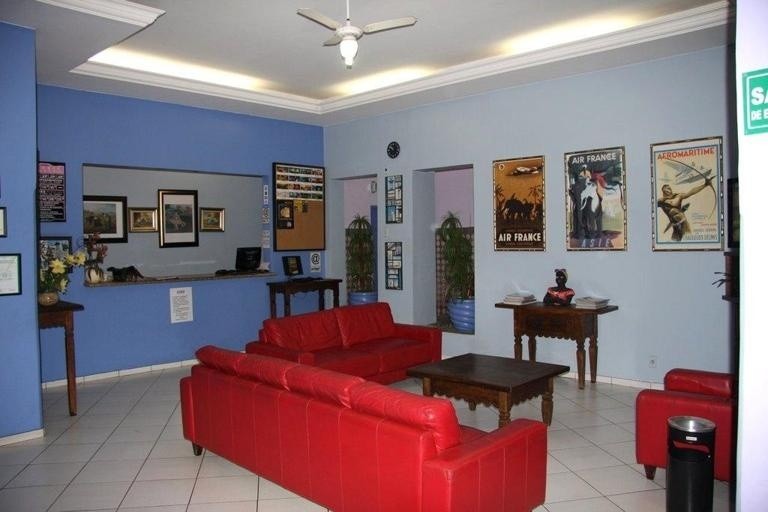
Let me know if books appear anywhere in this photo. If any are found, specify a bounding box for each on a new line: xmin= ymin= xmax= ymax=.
xmin=575 ymin=295 xmax=609 ymax=311
xmin=502 ymin=291 xmax=539 ymax=306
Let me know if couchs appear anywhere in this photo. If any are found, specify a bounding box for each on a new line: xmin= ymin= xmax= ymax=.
xmin=245 ymin=301 xmax=442 ymax=385
xmin=635 ymin=369 xmax=737 ymax=480
xmin=179 ymin=346 xmax=547 ymax=512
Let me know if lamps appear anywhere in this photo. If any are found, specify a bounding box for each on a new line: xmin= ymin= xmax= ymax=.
xmin=339 ymin=39 xmax=358 ymax=66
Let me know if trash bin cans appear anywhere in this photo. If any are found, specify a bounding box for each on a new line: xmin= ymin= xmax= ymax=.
xmin=666 ymin=415 xmax=717 ymax=512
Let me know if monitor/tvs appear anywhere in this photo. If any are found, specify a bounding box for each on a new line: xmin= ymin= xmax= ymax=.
xmin=235 ymin=247 xmax=261 ymax=273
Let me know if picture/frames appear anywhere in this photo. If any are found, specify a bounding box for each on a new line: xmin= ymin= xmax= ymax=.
xmin=129 ymin=206 xmax=159 ymax=233
xmin=0 ymin=254 xmax=22 ymax=296
xmin=40 ymin=237 xmax=73 ymax=274
xmin=83 ymin=195 xmax=128 ymax=243
xmin=649 ymin=135 xmax=725 ymax=253
xmin=727 ymin=177 xmax=740 ymax=249
xmin=158 ymin=188 xmax=199 ymax=247
xmin=0 ymin=207 xmax=7 ymax=237
xmin=491 ymin=156 xmax=547 ymax=252
xmin=272 ymin=161 xmax=326 ymax=251
xmin=199 ymin=207 xmax=225 ymax=233
xmin=563 ymin=145 xmax=628 ymax=252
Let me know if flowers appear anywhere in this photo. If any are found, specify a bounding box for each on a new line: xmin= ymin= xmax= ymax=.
xmin=84 ymin=232 xmax=108 ymax=281
xmin=39 ymin=253 xmax=86 ymax=294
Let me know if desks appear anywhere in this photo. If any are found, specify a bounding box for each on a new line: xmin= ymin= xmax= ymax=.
xmin=38 ymin=300 xmax=84 ymax=416
xmin=265 ymin=277 xmax=343 ymax=320
xmin=494 ymin=302 xmax=619 ymax=390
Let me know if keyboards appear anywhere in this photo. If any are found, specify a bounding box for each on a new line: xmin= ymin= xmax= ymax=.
xmin=228 ymin=270 xmax=236 ymax=273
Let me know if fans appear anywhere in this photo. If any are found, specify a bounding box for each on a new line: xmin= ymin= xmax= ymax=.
xmin=295 ymin=1 xmax=416 ymax=46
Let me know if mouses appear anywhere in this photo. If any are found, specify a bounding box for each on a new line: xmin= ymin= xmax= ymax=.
xmin=215 ymin=269 xmax=227 ymax=274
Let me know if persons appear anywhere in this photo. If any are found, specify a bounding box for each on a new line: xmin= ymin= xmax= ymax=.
xmin=542 ymin=268 xmax=575 ymax=306
xmin=656 ymin=181 xmax=711 ymax=241
xmin=580 ymin=163 xmax=590 ymax=181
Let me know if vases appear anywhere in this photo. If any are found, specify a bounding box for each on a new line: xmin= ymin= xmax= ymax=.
xmin=39 ymin=288 xmax=59 ymax=306
xmin=85 ymin=266 xmax=103 ymax=283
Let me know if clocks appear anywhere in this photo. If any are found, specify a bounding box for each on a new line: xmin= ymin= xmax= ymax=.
xmin=387 ymin=142 xmax=399 ymax=158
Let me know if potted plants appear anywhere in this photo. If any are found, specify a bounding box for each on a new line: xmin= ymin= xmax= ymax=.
xmin=440 ymin=212 xmax=474 ymax=334
xmin=346 ymin=214 xmax=377 ymax=305
